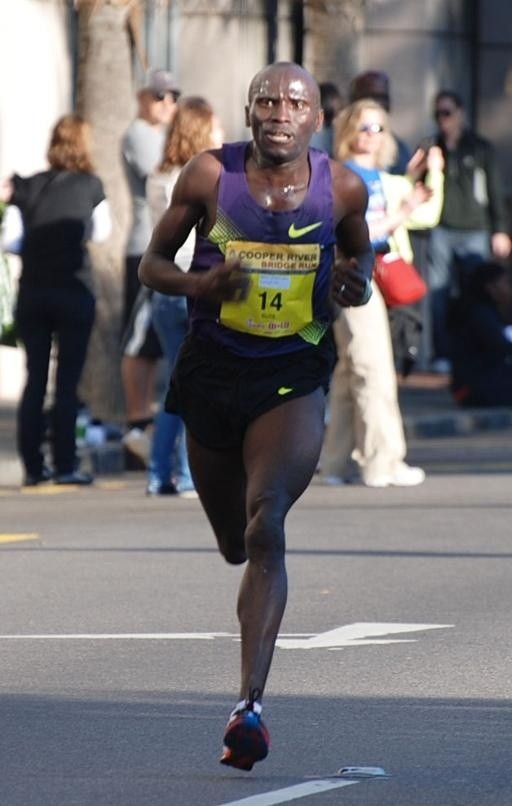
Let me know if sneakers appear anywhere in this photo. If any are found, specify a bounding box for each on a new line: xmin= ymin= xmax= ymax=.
xmin=217 ymin=684 xmax=269 ymax=772
xmin=56 ymin=473 xmax=93 ymax=484
xmin=23 ymin=466 xmax=55 ymax=485
xmin=361 ymin=463 xmax=426 ymax=488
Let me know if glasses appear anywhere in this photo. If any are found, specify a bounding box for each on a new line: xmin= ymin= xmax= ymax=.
xmin=436 ymin=110 xmax=450 ymax=118
xmin=359 ymin=123 xmax=383 ymax=134
xmin=155 ymin=90 xmax=180 ymax=102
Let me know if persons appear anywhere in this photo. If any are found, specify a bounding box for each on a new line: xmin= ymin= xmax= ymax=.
xmin=0 ymin=112 xmax=117 ymax=486
xmin=118 ymin=68 xmax=510 ymax=489
xmin=142 ymin=57 xmax=378 ymax=768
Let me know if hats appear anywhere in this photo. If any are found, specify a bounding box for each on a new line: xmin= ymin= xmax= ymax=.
xmin=137 ymin=68 xmax=180 ymax=94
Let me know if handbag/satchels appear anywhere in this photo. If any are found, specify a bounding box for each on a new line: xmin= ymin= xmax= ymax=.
xmin=371 ymin=249 xmax=427 ymax=308
